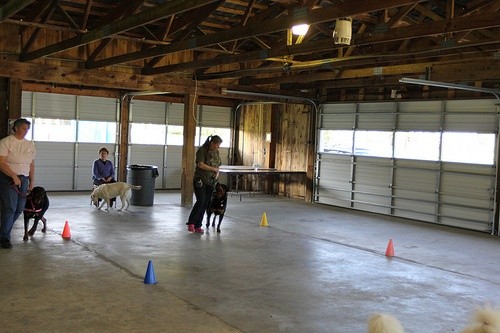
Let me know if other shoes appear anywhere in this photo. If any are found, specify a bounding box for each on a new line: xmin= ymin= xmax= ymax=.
xmin=0 ymin=238 xmax=13 ymax=249
xmin=195 ymin=227 xmax=204 ymax=233
xmin=188 ymin=224 xmax=194 ymax=232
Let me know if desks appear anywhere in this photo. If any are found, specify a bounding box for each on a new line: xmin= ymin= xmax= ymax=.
xmin=216 ymin=166 xmax=307 ymax=201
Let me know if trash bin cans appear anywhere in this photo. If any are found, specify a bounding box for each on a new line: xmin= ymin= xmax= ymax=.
xmin=127 ymin=164 xmax=158 ymax=206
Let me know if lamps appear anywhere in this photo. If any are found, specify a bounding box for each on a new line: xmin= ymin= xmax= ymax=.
xmin=334 ymin=17 xmax=352 ymax=45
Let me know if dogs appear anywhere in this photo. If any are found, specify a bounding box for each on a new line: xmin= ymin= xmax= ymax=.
xmin=23 ymin=186 xmax=49 ymax=241
xmin=91 ymin=182 xmax=143 ymax=212
xmin=205 ymin=183 xmax=231 ymax=233
xmin=368 ymin=308 xmax=500 ymax=333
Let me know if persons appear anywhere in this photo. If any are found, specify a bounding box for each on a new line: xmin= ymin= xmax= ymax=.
xmin=186 ymin=134 xmax=222 ymax=232
xmin=0 ymin=118 xmax=36 ymax=248
xmin=92 ymin=148 xmax=116 ymax=207
